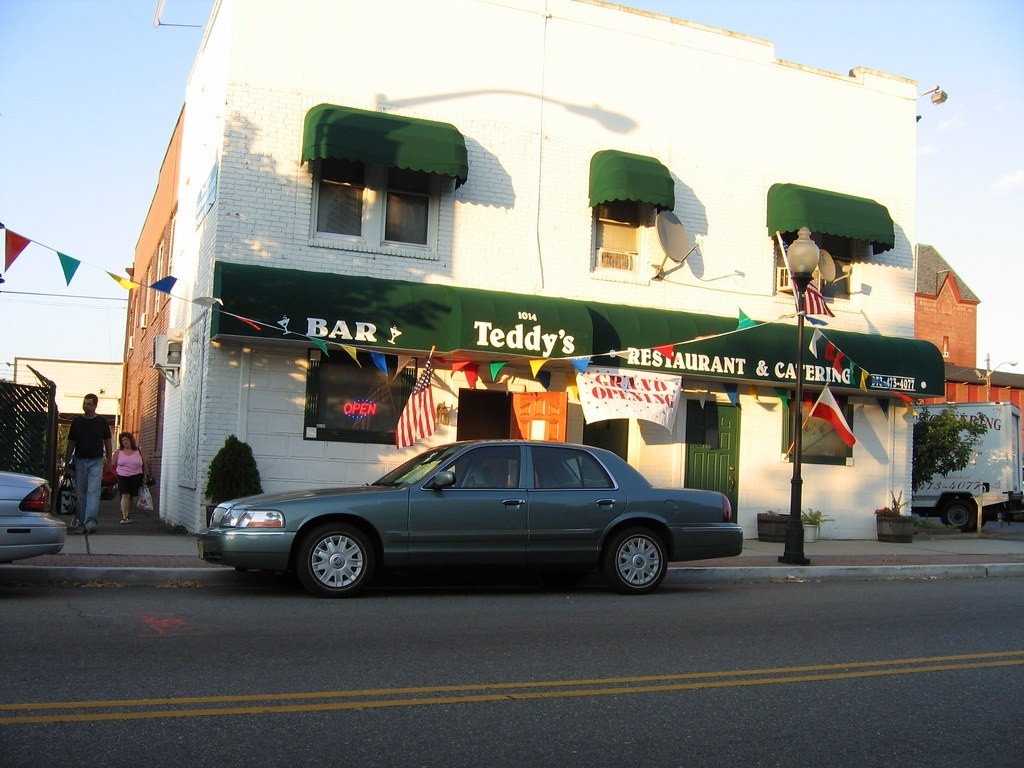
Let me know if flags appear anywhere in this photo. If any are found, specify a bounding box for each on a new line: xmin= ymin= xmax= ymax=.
xmin=395 ymin=357 xmax=437 ymax=449
xmin=782 ymin=239 xmax=835 ymax=318
xmin=808 ymin=386 xmax=856 ymax=448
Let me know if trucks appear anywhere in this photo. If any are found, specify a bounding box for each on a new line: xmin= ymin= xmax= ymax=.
xmin=912 ymin=400 xmax=1024 ymax=533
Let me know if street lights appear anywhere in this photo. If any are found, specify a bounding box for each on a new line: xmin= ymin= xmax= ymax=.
xmin=771 ymin=226 xmax=822 ymax=564
xmin=973 ymin=352 xmax=1019 ymax=403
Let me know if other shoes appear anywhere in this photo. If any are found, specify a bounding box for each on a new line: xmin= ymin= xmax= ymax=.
xmin=75 ymin=525 xmax=83 ymax=533
xmin=121 ymin=518 xmax=126 ymax=523
xmin=127 ymin=518 xmax=132 ymax=523
xmin=86 ymin=521 xmax=97 ymax=533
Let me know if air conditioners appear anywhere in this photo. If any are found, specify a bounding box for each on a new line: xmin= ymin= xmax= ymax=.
xmin=141 ymin=313 xmax=148 ymax=329
xmin=129 ymin=337 xmax=134 ymax=349
xmin=596 ymin=249 xmax=631 ymax=271
xmin=152 ymin=334 xmax=179 ymax=366
xmin=777 ymin=267 xmax=821 ymax=294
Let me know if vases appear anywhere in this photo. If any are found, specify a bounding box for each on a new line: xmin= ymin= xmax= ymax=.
xmin=876 ymin=516 xmax=914 ymax=544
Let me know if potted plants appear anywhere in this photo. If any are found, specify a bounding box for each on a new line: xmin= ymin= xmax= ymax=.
xmin=801 ymin=508 xmax=835 ymax=544
xmin=757 ymin=511 xmax=790 ymax=543
xmin=204 ymin=433 xmax=265 ymax=528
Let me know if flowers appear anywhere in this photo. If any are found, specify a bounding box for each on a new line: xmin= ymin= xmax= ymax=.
xmin=874 ymin=489 xmax=909 ymax=518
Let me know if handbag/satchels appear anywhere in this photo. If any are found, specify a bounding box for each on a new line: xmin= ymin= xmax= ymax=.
xmin=145 ymin=475 xmax=156 ymax=487
xmin=137 ymin=485 xmax=154 ymax=511
xmin=56 ymin=473 xmax=77 ymax=515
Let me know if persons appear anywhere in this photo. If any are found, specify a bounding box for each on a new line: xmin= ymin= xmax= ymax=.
xmin=64 ymin=394 xmax=112 ymax=532
xmin=112 ymin=432 xmax=147 ymax=523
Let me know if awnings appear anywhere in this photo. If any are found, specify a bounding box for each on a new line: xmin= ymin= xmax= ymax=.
xmin=301 ymin=104 xmax=468 ymax=192
xmin=767 ymin=183 xmax=895 ymax=256
xmin=210 ymin=261 xmax=944 ymax=397
xmin=588 ymin=150 xmax=675 ymax=215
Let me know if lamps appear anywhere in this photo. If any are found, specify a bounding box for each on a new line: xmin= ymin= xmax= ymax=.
xmin=916 ymin=85 xmax=948 ymax=107
xmin=437 ymin=401 xmax=450 ymax=425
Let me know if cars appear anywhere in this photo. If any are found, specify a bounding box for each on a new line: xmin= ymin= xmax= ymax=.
xmin=0 ymin=468 xmax=68 ymax=564
xmin=196 ymin=438 xmax=745 ymax=599
